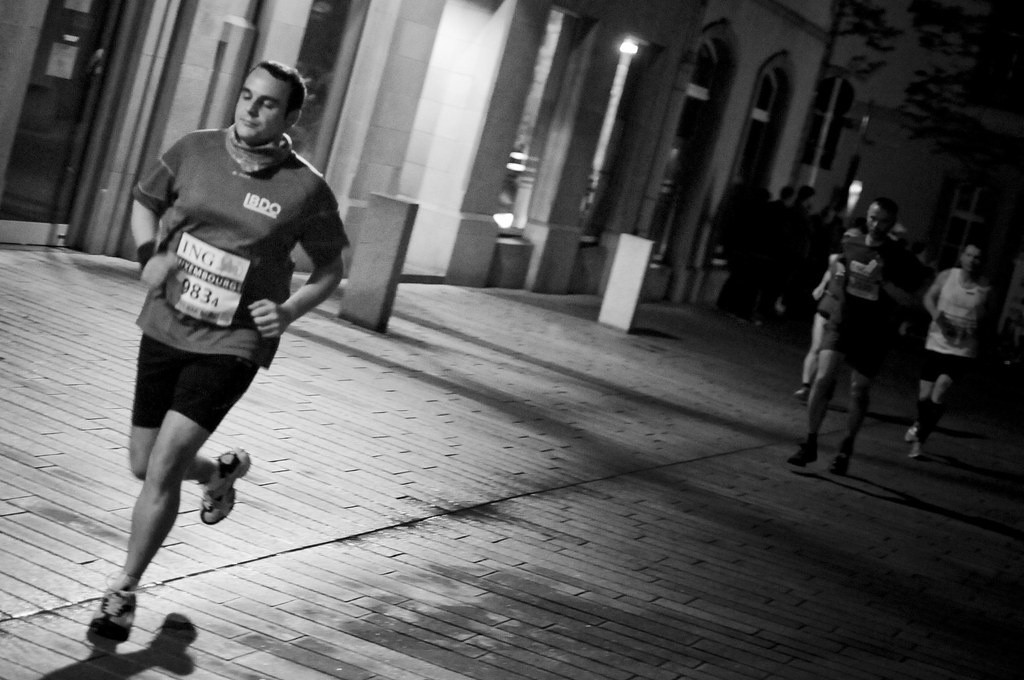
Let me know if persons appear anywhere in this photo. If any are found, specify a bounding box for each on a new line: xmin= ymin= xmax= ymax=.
xmin=88 ymin=60 xmax=352 ymax=642
xmin=720 ymin=185 xmax=995 ymax=477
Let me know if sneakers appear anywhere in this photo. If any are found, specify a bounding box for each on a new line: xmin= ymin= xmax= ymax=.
xmin=199 ymin=447 xmax=251 ymax=525
xmin=89 ymin=573 xmax=137 ymax=642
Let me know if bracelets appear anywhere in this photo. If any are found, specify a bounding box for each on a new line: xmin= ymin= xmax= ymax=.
xmin=137 ymin=240 xmax=155 ymax=270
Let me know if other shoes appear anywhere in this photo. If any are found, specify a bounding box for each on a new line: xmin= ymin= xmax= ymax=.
xmin=734 ymin=309 xmax=768 ymax=326
xmin=794 ymin=382 xmax=812 ymax=398
xmin=908 ymin=441 xmax=925 ymax=457
xmin=904 ymin=423 xmax=920 ymax=442
xmin=828 ymin=452 xmax=850 ymax=476
xmin=786 ymin=446 xmax=820 ymax=467
